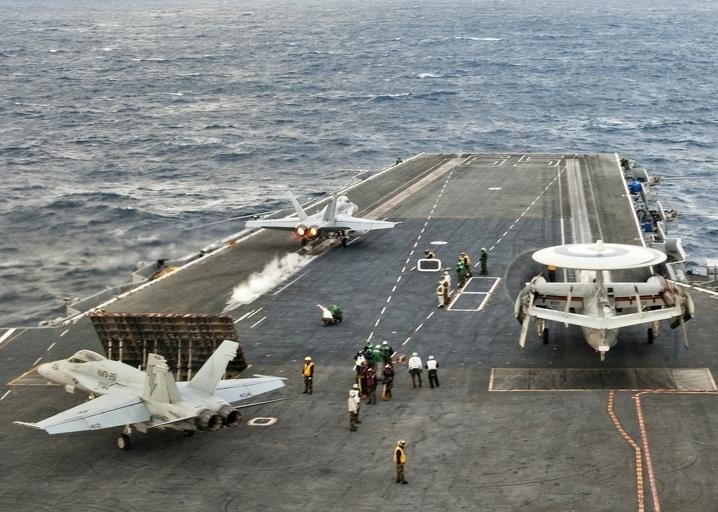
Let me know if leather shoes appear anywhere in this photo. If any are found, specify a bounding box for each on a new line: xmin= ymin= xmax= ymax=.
xmin=309 ymin=391 xmax=312 ymax=394
xmin=350 ymin=428 xmax=356 ymax=431
xmin=355 ymin=421 xmax=361 ymax=424
xmin=302 ymin=391 xmax=307 ymax=393
xmin=402 ymin=481 xmax=407 ymax=484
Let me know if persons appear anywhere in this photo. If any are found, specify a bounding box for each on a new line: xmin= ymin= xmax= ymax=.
xmin=329 ymin=305 xmax=343 ymax=323
xmin=302 ymin=356 xmax=314 ymax=395
xmin=347 ymin=340 xmax=395 ymax=432
xmin=394 ymin=439 xmax=408 ymax=484
xmin=437 ymin=252 xmax=471 ymax=308
xmin=316 ymin=304 xmax=335 ymax=327
xmin=424 ymin=355 xmax=440 ymax=388
xmin=545 ymin=265 xmax=556 ymax=282
xmin=407 ymin=352 xmax=423 ymax=388
xmin=424 ymin=250 xmax=435 ymax=259
xmin=479 ymin=248 xmax=487 ymax=272
xmin=396 ymin=157 xmax=403 ymax=164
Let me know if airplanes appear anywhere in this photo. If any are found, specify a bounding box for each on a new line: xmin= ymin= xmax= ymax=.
xmin=512 ymin=242 xmax=694 ymax=362
xmin=13 ymin=337 xmax=285 ymax=451
xmin=245 ymin=191 xmax=403 ymax=245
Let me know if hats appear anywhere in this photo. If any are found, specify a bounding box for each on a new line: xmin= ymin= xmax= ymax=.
xmin=428 ymin=355 xmax=434 ymax=360
xmin=349 ymin=390 xmax=355 ymax=397
xmin=352 ymin=384 xmax=359 ymax=390
xmin=366 ymin=368 xmax=374 ymax=373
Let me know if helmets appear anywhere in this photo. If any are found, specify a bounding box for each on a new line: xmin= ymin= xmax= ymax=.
xmin=441 ymin=248 xmax=485 ymax=287
xmin=304 ymin=357 xmax=311 ymax=361
xmin=397 ymin=441 xmax=405 ymax=447
xmin=375 ymin=339 xmax=388 ymax=349
